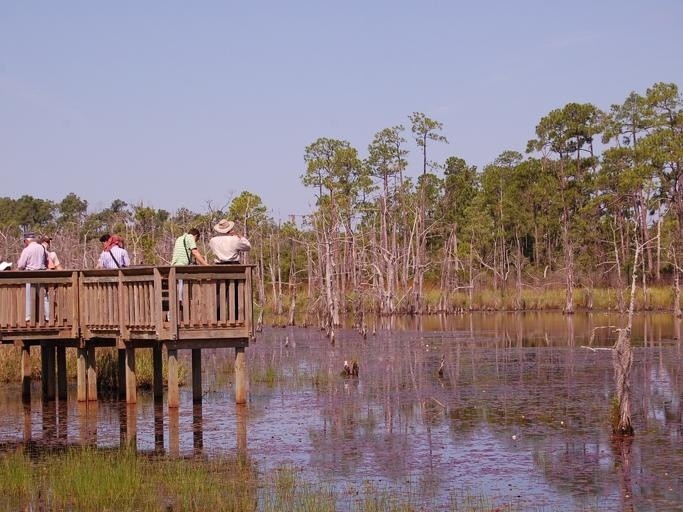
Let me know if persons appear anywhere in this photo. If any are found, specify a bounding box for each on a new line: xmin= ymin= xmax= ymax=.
xmin=0 ymin=260 xmax=13 ymax=271
xmin=15 ymin=231 xmax=51 ymax=323
xmin=167 ymin=227 xmax=210 ymax=323
xmin=95 ymin=234 xmax=110 ymax=269
xmin=39 ymin=234 xmax=63 ymax=271
xmin=208 ymin=220 xmax=252 ymax=321
xmin=99 ymin=235 xmax=131 ymax=269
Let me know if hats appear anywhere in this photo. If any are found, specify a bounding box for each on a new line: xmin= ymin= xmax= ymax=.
xmin=214 ymin=218 xmax=235 ymax=234
xmin=23 ymin=232 xmax=35 ymax=239
